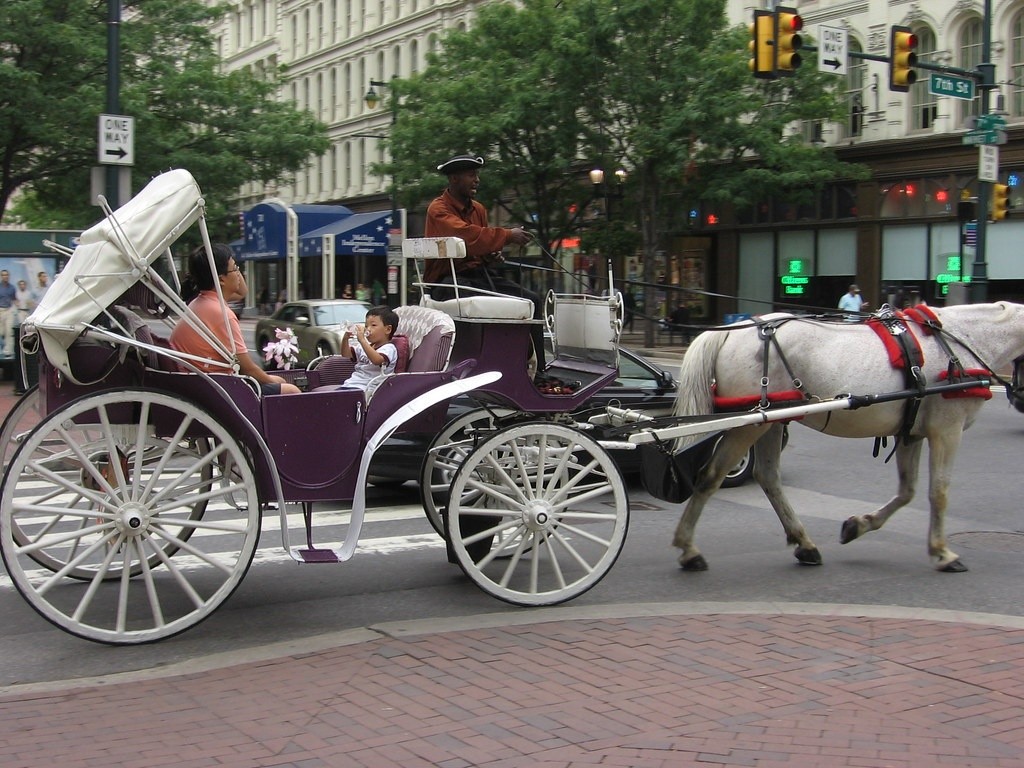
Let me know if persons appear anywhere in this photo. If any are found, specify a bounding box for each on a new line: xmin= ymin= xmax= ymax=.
xmin=341 ymin=278 xmax=387 ymax=306
xmin=0 ymin=268 xmax=51 ymax=358
xmin=623 ymin=285 xmax=636 ymax=331
xmin=424 ymin=156 xmax=546 ymax=375
xmin=279 ymin=282 xmax=304 ymax=305
xmin=258 ymin=287 xmax=270 ymax=314
xmin=167 ymin=242 xmax=300 ymax=395
xmin=312 ymin=305 xmax=399 ymax=392
xmin=838 ymin=285 xmax=869 ymax=322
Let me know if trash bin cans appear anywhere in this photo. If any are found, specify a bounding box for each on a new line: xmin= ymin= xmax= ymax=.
xmin=12 ymin=322 xmax=39 ymax=395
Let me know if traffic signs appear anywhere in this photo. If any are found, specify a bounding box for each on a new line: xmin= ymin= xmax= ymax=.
xmin=98 ymin=113 xmax=135 ymax=166
xmin=817 ymin=25 xmax=848 ymax=76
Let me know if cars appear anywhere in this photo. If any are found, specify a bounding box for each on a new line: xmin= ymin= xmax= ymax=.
xmin=366 ymin=341 xmax=789 ymax=492
xmin=255 ymin=299 xmax=375 ymax=369
xmin=227 ymin=300 xmax=245 ymax=320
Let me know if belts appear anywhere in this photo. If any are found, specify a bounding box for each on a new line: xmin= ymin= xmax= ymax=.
xmin=19 ymin=309 xmax=29 ymax=311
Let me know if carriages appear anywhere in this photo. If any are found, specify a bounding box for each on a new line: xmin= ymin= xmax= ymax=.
xmin=0 ymin=167 xmax=1022 ymax=646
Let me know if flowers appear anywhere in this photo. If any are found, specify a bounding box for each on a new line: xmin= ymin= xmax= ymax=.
xmin=262 ymin=328 xmax=309 ymax=372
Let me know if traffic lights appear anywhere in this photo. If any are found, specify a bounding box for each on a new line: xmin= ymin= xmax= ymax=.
xmin=958 ymin=199 xmax=978 ymax=221
xmin=889 ymin=25 xmax=918 ymax=93
xmin=747 ymin=9 xmax=774 ymax=80
xmin=772 ymin=5 xmax=803 ymax=78
xmin=991 ymin=183 xmax=1011 ymax=221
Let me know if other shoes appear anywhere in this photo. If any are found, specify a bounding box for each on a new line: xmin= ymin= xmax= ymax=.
xmin=15 ymin=388 xmax=24 ymax=396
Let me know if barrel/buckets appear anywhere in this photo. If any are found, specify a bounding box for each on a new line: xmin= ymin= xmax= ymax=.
xmin=439 ymin=507 xmax=504 ymax=566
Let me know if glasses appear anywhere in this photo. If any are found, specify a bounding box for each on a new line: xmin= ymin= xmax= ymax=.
xmin=219 ymin=263 xmax=240 ymax=277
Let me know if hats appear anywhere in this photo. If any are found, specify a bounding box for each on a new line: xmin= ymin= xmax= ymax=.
xmin=437 ymin=155 xmax=485 ymax=173
xmin=849 ymin=284 xmax=861 ymax=292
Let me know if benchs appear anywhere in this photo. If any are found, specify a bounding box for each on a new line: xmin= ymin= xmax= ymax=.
xmin=402 ymin=237 xmax=546 ymax=391
xmin=313 ymin=305 xmax=456 ymax=387
xmin=89 ymin=304 xmax=160 ymax=370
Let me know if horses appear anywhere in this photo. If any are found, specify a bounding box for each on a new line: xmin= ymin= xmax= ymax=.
xmin=671 ymin=301 xmax=1024 ymax=573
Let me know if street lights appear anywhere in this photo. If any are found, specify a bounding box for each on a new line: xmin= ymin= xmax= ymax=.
xmin=363 ymin=80 xmax=401 ymax=307
xmin=589 ymin=169 xmax=627 ymax=286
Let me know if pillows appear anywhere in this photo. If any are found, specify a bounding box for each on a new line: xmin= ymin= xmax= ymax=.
xmin=151 ymin=332 xmax=185 ymax=372
xmin=390 ymin=334 xmax=411 ymax=373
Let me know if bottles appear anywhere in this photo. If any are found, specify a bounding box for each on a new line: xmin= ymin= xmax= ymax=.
xmin=339 ymin=319 xmax=370 ymax=338
